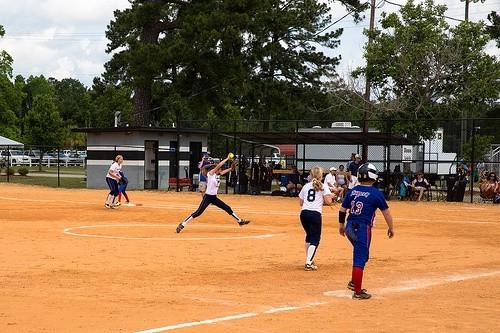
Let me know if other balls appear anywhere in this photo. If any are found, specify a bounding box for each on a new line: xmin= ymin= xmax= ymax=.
xmin=229 ymin=152 xmax=234 ymax=159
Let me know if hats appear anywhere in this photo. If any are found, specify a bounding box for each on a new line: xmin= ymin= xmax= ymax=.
xmin=205 ymin=154 xmax=209 ymax=158
xmin=355 ymin=154 xmax=361 ymax=158
xmin=329 ymin=167 xmax=337 ymax=171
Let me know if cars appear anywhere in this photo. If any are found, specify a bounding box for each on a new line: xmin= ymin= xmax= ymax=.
xmin=0 ymin=148 xmax=88 ymax=167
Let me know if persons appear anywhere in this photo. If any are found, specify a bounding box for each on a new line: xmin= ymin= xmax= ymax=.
xmin=399 ymin=171 xmax=431 ymax=201
xmin=198 ymin=154 xmax=211 ymax=201
xmin=116 ymin=170 xmax=130 ymax=205
xmin=339 ymin=163 xmax=395 ymax=300
xmin=275 ymin=152 xmax=366 ymax=207
xmin=482 ymin=172 xmax=499 ymax=202
xmin=105 ymin=155 xmax=123 ymax=209
xmin=175 ymin=154 xmax=251 ymax=233
xmin=297 ymin=166 xmax=332 ymax=271
xmin=460 ymin=158 xmax=488 ymax=183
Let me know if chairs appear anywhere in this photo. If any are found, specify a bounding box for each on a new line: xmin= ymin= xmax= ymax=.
xmin=389 ymin=176 xmax=432 ymax=202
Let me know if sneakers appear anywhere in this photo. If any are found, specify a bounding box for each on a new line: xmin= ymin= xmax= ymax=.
xmin=175 ymin=223 xmax=184 ymax=234
xmin=238 ymin=219 xmax=250 ymax=226
xmin=305 ymin=263 xmax=317 ymax=271
xmin=347 ymin=281 xmax=355 ymax=291
xmin=352 ymin=289 xmax=371 ymax=299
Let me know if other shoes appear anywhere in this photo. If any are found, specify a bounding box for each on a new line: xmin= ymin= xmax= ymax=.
xmin=116 ymin=202 xmax=121 ymax=205
xmin=106 ymin=204 xmax=110 ymax=208
xmin=112 ymin=205 xmax=118 ymax=209
xmin=124 ymin=202 xmax=130 ymax=204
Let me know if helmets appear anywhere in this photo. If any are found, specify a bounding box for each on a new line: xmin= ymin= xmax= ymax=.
xmin=357 ymin=163 xmax=378 ymax=182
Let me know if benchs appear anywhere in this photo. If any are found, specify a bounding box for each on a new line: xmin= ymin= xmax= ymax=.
xmin=168 ymin=177 xmax=191 ymax=191
